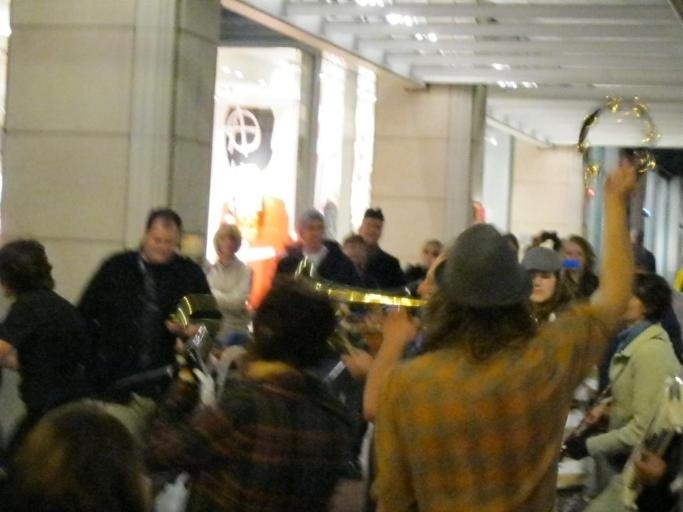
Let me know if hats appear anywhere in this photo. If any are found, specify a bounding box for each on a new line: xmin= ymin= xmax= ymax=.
xmin=522 ymin=246 xmax=564 ymax=272
xmin=434 ymin=224 xmax=532 ymax=308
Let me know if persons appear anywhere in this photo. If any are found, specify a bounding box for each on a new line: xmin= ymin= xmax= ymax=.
xmin=0 ymin=207 xmax=683 ymax=512
xmin=370 ymin=156 xmax=638 ymax=511
